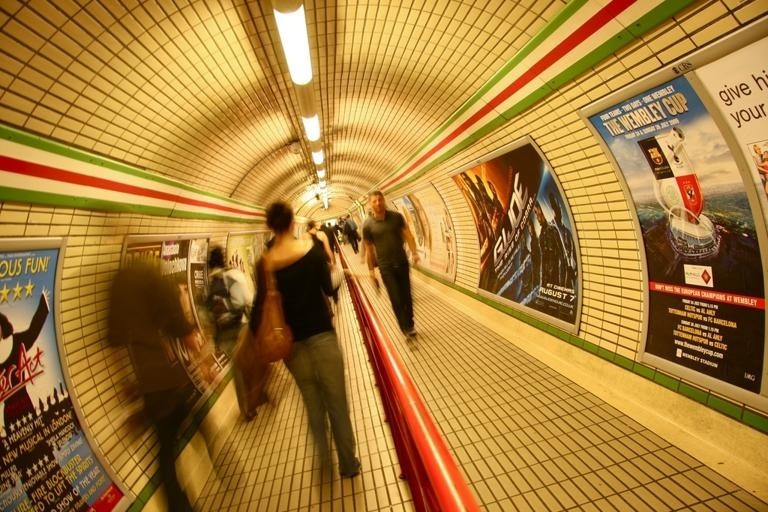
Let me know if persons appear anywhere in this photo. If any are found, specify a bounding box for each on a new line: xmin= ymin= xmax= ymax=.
xmin=103 ymin=191 xmax=423 ymax=512
xmin=753 ymin=145 xmax=768 ymax=198
xmin=460 ymin=172 xmax=578 ymax=324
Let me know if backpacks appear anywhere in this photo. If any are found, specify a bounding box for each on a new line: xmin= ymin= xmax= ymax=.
xmin=207 ymin=268 xmax=244 ymax=329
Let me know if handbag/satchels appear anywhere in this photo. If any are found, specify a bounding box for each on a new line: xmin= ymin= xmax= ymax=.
xmin=255 ymin=292 xmax=292 ymax=361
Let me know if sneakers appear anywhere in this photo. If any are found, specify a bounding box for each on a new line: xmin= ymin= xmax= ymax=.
xmin=406 ymin=327 xmax=417 ymax=336
